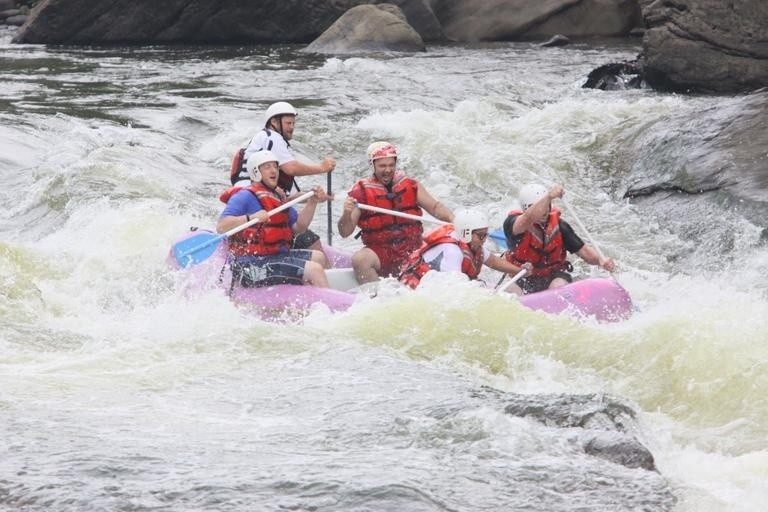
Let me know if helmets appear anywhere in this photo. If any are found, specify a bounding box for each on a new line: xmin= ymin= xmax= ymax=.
xmin=452 ymin=208 xmax=489 ymax=244
xmin=518 ymin=184 xmax=548 ymax=213
xmin=366 ymin=141 xmax=396 ymax=174
xmin=264 ymin=101 xmax=297 ymax=128
xmin=247 ymin=149 xmax=280 ymax=182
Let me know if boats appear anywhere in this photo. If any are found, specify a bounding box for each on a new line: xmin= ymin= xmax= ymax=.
xmin=167 ymin=226 xmax=632 ymax=330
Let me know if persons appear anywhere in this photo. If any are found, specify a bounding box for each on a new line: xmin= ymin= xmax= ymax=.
xmin=338 ymin=142 xmax=457 ymax=283
xmin=217 ymin=151 xmax=328 ymax=290
xmin=501 ymin=184 xmax=615 ymax=296
xmin=399 ymin=206 xmax=534 ymax=291
xmin=229 ymin=102 xmax=336 ymax=267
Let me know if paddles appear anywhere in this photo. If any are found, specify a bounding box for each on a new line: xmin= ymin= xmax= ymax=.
xmin=355 ymin=202 xmax=508 ymax=248
xmin=173 ymin=190 xmax=315 ymax=269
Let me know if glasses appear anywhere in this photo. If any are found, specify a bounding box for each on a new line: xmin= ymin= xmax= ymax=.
xmin=475 ymin=231 xmax=488 ymax=241
